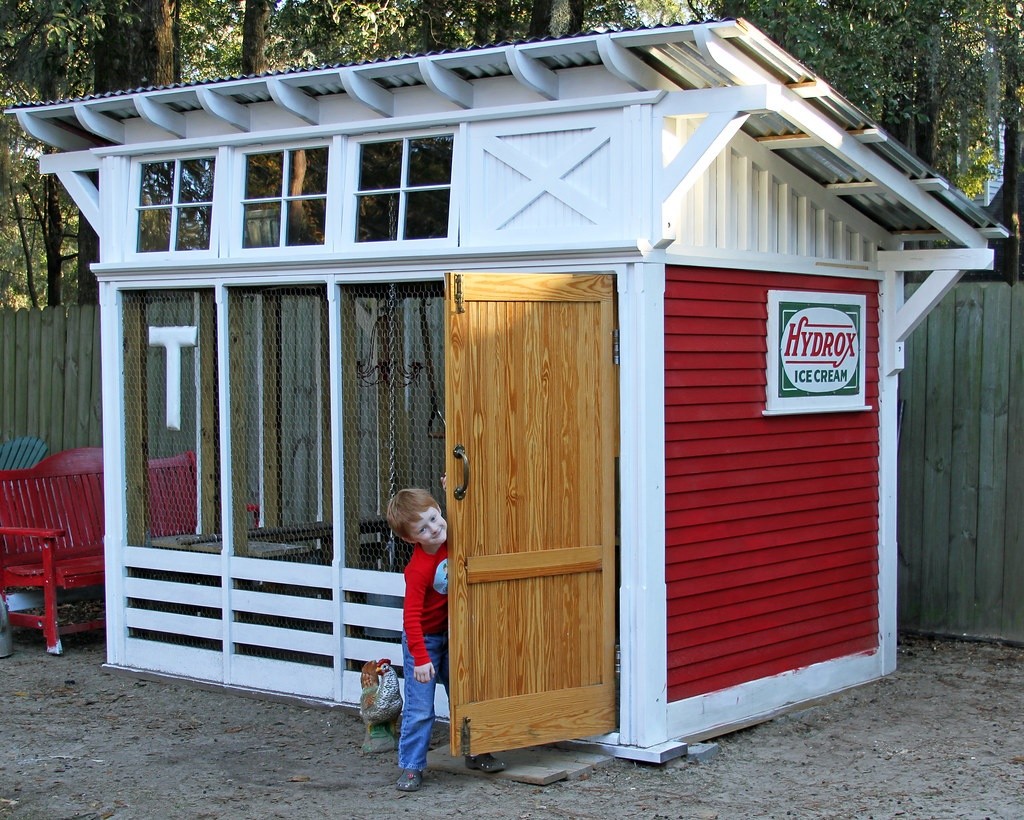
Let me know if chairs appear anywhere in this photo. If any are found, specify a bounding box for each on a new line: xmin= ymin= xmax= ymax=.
xmin=0 ymin=435 xmax=47 ymax=471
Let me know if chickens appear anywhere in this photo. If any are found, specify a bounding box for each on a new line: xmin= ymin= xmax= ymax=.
xmin=359 ymin=658 xmax=405 ymax=758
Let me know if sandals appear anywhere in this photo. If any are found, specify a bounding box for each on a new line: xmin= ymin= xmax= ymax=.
xmin=395 ymin=768 xmax=423 ymax=791
xmin=465 ymin=753 xmax=505 ymax=772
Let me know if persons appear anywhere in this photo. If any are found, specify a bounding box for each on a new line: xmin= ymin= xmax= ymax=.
xmin=387 ymin=472 xmax=506 ymax=791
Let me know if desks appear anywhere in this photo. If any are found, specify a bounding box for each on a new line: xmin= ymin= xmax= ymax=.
xmin=152 ymin=538 xmax=309 ymax=659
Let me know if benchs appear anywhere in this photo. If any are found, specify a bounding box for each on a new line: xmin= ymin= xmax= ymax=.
xmin=0 ymin=447 xmax=259 ymax=656
